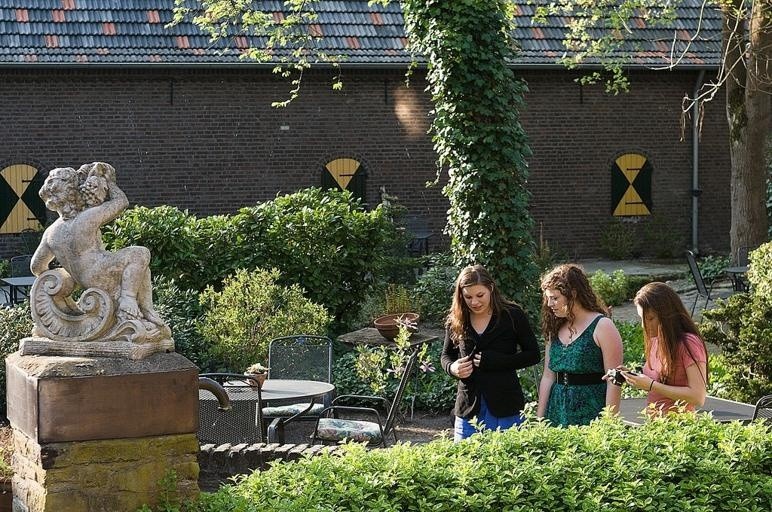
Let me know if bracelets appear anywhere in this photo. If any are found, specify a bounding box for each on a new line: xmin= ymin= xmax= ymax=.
xmin=649 ymin=379 xmax=656 ymax=392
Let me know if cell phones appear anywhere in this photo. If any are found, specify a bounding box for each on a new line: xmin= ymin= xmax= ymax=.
xmin=609 ymin=370 xmax=637 ymax=383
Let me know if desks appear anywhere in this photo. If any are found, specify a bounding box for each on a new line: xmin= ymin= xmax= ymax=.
xmin=199 ymin=379 xmax=334 ymax=443
xmin=338 ymin=327 xmax=439 ymax=420
xmin=722 ymin=265 xmax=750 ymax=292
xmin=410 ymin=232 xmax=435 ymax=275
xmin=1 ymin=275 xmax=33 ymax=304
xmin=310 ymin=343 xmax=420 ymax=448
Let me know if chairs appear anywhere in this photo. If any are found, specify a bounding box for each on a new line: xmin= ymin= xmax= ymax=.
xmin=21 ymin=228 xmax=38 ymax=253
xmin=751 ymin=394 xmax=772 ymax=424
xmin=681 ymin=249 xmax=736 ymax=324
xmin=10 ymin=254 xmax=31 ymax=306
xmin=197 ymin=372 xmax=263 ymax=445
xmin=262 ymin=335 xmax=339 ymax=444
xmin=409 ymin=219 xmax=429 ymax=256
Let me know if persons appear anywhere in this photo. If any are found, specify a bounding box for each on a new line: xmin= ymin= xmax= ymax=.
xmin=603 ymin=280 xmax=708 ymax=422
xmin=30 ymin=161 xmax=170 ymax=327
xmin=536 ymin=264 xmax=624 ymax=430
xmin=442 ymin=264 xmax=541 ymax=444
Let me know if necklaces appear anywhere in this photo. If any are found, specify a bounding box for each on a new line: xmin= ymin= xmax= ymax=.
xmin=565 ymin=316 xmax=594 ymax=342
